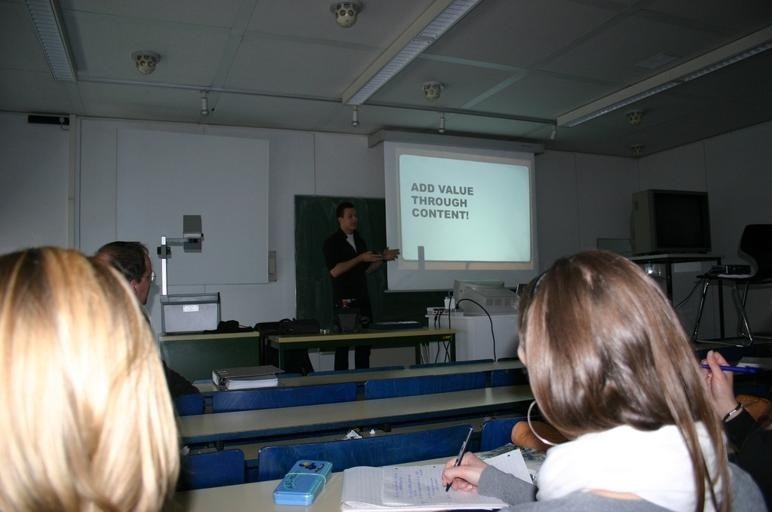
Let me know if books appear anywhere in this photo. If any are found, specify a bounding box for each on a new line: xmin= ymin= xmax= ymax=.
xmin=212 ymin=364 xmax=285 ymax=390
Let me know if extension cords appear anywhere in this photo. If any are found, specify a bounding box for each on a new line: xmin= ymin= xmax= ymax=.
xmin=449 ymin=309 xmax=464 ymax=316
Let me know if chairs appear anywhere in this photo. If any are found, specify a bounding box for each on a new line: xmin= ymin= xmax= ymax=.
xmin=689 ymin=223 xmax=772 ymax=349
xmin=177 ymin=358 xmax=541 ymax=494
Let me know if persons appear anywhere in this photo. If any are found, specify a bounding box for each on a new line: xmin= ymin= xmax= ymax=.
xmin=0 ymin=245 xmax=185 ymax=512
xmin=97 ymin=241 xmax=202 ymax=400
xmin=699 ymin=349 xmax=772 ymax=512
xmin=442 ymin=249 xmax=771 ymax=512
xmin=322 ymin=202 xmax=400 ymax=372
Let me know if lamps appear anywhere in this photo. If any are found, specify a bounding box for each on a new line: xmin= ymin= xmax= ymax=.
xmin=340 ymin=1 xmax=483 ymax=107
xmin=439 ymin=112 xmax=446 ymax=133
xmin=352 ymin=104 xmax=359 ymax=128
xmin=24 ymin=0 xmax=77 ymax=82
xmin=200 ymin=90 xmax=209 ymax=115
xmin=557 ymin=25 xmax=771 ymax=128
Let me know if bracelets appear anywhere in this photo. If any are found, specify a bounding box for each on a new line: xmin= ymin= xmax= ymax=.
xmin=722 ymin=401 xmax=740 ymax=423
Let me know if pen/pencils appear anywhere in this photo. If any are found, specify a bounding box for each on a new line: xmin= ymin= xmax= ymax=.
xmin=700 ymin=364 xmax=756 ymax=374
xmin=446 ymin=428 xmax=474 ymax=492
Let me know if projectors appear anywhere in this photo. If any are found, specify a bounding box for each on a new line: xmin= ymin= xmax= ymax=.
xmin=452 ymin=279 xmax=521 ymax=316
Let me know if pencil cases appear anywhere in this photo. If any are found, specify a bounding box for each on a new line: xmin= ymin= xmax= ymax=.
xmin=273 ymin=459 xmax=333 ymax=506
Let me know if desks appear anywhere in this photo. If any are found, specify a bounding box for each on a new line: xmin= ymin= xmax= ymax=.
xmin=175 ymin=452 xmax=540 ymax=512
xmin=626 ymin=254 xmax=725 ymax=341
xmin=267 ymin=327 xmax=460 ymax=374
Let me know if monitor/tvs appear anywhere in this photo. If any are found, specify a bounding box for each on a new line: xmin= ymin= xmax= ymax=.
xmin=630 ymin=188 xmax=712 ymax=257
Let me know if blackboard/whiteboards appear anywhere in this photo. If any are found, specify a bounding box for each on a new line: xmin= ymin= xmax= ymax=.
xmin=295 ymin=194 xmax=528 ymax=327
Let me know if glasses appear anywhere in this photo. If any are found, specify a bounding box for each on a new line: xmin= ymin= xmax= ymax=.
xmin=136 ymin=271 xmax=157 ymax=284
